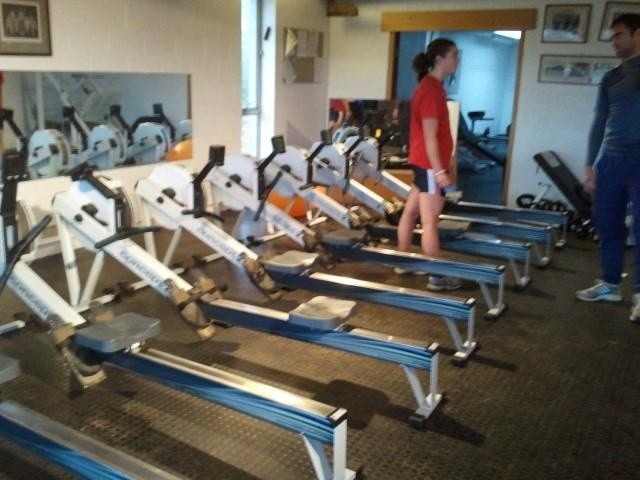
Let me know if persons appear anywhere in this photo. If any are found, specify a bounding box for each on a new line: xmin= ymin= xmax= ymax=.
xmin=394 ymin=37 xmax=465 ymax=292
xmin=328 ymin=100 xmax=345 ymax=133
xmin=575 ymin=12 xmax=640 ymax=322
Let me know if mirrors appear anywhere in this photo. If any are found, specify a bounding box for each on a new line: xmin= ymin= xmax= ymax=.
xmin=1 ymin=71 xmax=193 ymax=179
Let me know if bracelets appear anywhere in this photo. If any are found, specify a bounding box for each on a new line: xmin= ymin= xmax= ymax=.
xmin=433 ymin=169 xmax=446 ymax=176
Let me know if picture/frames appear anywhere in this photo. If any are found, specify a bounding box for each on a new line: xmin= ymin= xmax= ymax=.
xmin=538 ymin=55 xmax=619 ymax=85
xmin=542 ymin=5 xmax=592 ymax=44
xmin=599 ymin=1 xmax=639 ymax=41
xmin=1 ymin=1 xmax=51 ymax=56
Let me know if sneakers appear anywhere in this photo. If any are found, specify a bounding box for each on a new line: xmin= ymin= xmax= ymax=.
xmin=574 ymin=278 xmax=625 ymax=303
xmin=427 ymin=273 xmax=464 ymax=291
xmin=629 ymin=292 xmax=640 ymax=322
xmin=393 ymin=266 xmax=428 ymax=275
